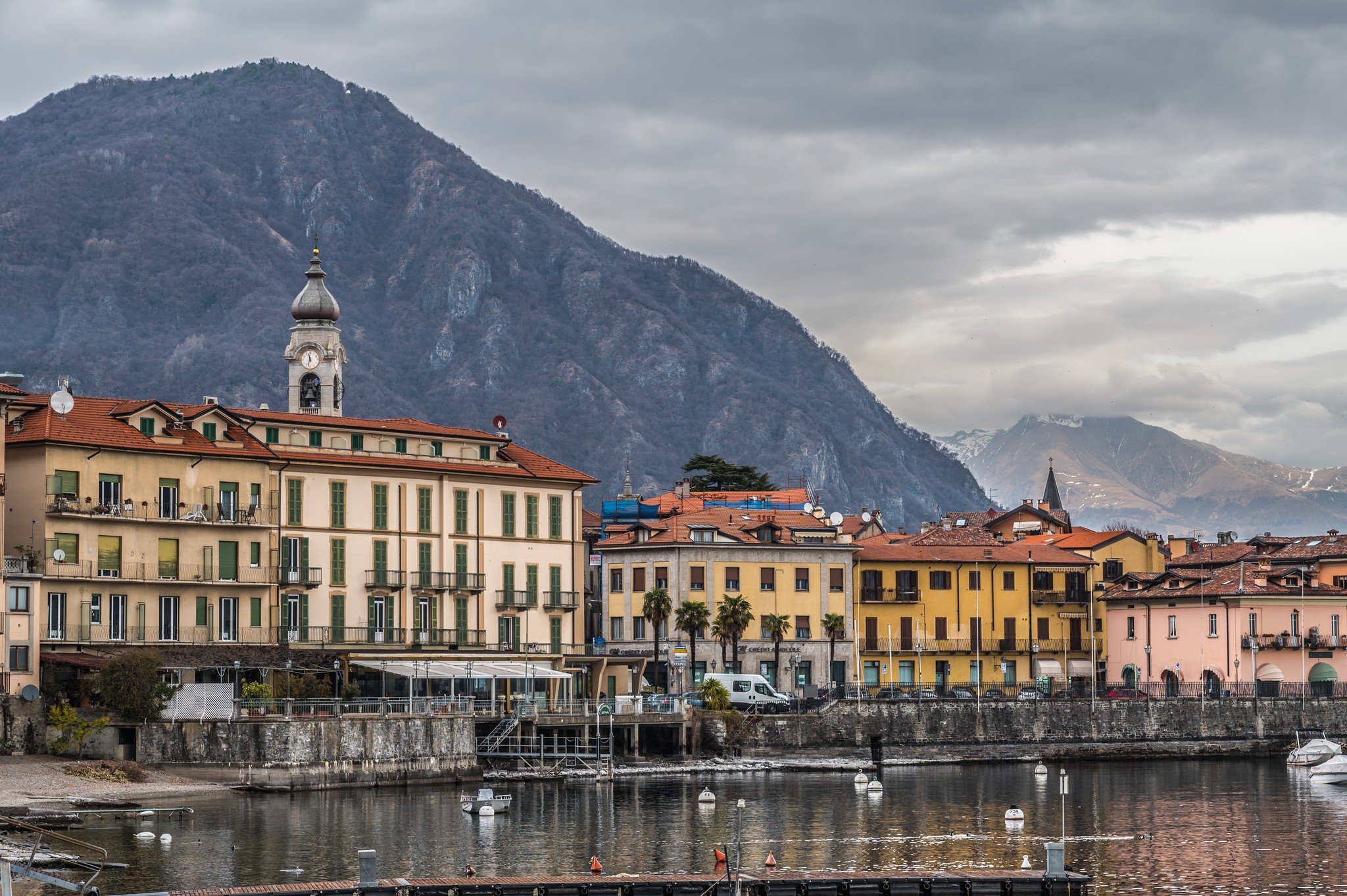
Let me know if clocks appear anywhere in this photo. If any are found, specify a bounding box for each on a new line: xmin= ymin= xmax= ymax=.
xmin=301 ymin=349 xmax=320 ymax=369
xmin=334 ymin=354 xmax=339 ymax=371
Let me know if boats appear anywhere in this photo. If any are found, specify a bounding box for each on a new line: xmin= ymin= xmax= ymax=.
xmin=461 ymin=789 xmax=513 ymax=812
xmin=1286 ymin=728 xmax=1347 ymax=785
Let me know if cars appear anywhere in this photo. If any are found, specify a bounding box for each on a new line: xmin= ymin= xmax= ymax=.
xmin=1101 ymin=685 xmax=1152 ymax=698
xmin=1056 ymin=689 xmax=1085 ymax=698
xmin=982 ymin=689 xmax=1006 ymax=698
xmin=938 ymin=686 xmax=978 ymax=699
xmin=679 ymin=691 xmax=703 ymax=706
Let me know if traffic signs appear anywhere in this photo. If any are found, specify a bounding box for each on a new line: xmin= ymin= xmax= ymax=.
xmin=674 ymin=646 xmax=688 ymax=666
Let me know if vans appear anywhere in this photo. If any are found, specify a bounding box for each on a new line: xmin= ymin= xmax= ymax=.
xmin=703 ymin=673 xmax=791 ymax=714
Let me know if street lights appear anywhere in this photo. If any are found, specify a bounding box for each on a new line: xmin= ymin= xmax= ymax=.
xmin=285 ymin=659 xmax=292 ymax=698
xmin=581 ymin=665 xmax=587 ymax=700
xmin=1234 ymin=659 xmax=1240 ymax=697
xmin=632 ymin=665 xmax=638 ymax=698
xmin=915 ymin=641 xmax=923 ymax=719
xmin=464 ymin=660 xmax=473 ymax=697
xmin=381 ymin=659 xmax=387 ymax=697
xmin=1032 ymin=643 xmax=1039 ymax=718
xmin=795 ymin=653 xmax=802 ymax=732
xmin=424 ymin=659 xmax=430 ymax=697
xmin=333 ymin=658 xmax=340 ymax=698
xmin=235 ymin=661 xmax=240 ymax=699
xmin=1144 ymin=643 xmax=1152 ymax=717
xmin=710 ymin=659 xmax=717 ymax=673
xmin=530 ymin=665 xmax=537 ymax=700
xmin=413 ymin=660 xmax=420 ymax=697
xmin=1252 ymin=643 xmax=1259 ymax=716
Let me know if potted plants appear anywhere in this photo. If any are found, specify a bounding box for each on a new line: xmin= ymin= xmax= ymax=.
xmin=240 ymin=678 xmax=273 ymax=719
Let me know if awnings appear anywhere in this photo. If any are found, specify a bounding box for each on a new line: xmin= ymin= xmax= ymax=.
xmin=1308 ymin=662 xmax=1338 ymax=682
xmin=1066 ymin=658 xmax=1095 ymax=677
xmin=1255 ymin=663 xmax=1284 ymax=682
xmin=1034 ymin=658 xmax=1062 ymax=677
xmin=40 ymin=652 xmax=120 ymax=670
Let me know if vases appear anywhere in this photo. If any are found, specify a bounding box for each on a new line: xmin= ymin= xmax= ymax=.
xmin=179 ymin=504 xmax=185 ymax=507
xmin=86 ymin=500 xmax=90 ymax=503
xmin=202 ymin=507 xmax=208 ymax=510
xmin=142 ymin=503 xmax=148 ymax=506
xmin=125 ymin=500 xmax=132 ymax=504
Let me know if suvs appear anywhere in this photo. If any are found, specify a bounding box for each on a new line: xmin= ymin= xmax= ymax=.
xmin=1018 ymin=686 xmax=1045 ymax=699
xmin=806 ymin=688 xmax=873 ymax=710
xmin=638 ymin=691 xmax=681 ymax=708
xmin=778 ymin=692 xmax=798 ymax=701
xmin=876 ymin=688 xmax=941 ymax=699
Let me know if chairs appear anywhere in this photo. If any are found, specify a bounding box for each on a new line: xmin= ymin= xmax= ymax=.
xmin=108 ymin=501 xmax=121 ymax=516
xmin=216 ymin=503 xmax=232 ymax=522
xmin=179 ymin=503 xmax=207 ymax=521
xmin=241 ymin=504 xmax=257 ymax=523
xmin=124 ymin=504 xmax=137 ymax=517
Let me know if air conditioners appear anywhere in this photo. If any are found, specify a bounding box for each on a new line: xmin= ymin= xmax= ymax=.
xmin=1097 ymin=583 xmax=1105 ymax=590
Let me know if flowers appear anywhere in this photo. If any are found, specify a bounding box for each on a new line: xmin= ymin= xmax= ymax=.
xmin=127 ymin=498 xmax=132 ymax=501
xmin=203 ymin=504 xmax=208 ymax=507
xmin=86 ymin=496 xmax=91 ymax=499
xmin=180 ymin=502 xmax=186 ymax=504
xmin=142 ymin=501 xmax=147 ymax=503
